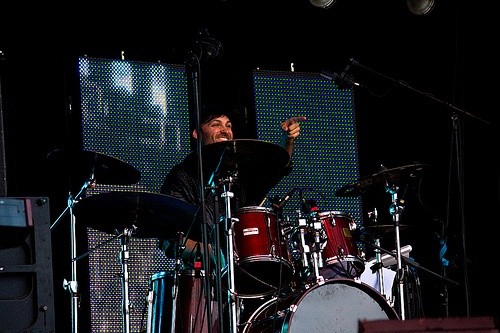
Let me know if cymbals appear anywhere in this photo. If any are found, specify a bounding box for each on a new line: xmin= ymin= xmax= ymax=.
xmin=335 ymin=164 xmax=425 ymax=197
xmin=45 ymin=148 xmax=141 ymax=185
xmin=182 ymin=139 xmax=290 ymax=181
xmin=72 ymin=191 xmax=195 ymax=238
xmin=368 ymin=225 xmax=409 ymax=232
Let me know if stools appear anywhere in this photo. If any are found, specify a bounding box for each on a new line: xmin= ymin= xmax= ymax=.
xmin=145 ymin=271 xmax=222 ymax=333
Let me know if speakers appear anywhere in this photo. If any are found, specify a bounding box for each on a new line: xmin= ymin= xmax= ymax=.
xmin=0 ymin=197 xmax=56 ymax=333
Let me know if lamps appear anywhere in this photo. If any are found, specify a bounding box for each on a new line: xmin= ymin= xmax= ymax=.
xmin=185 ymin=25 xmax=223 ymax=71
xmin=318 ymin=56 xmax=363 ymax=91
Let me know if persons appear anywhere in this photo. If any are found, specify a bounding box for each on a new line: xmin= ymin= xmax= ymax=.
xmin=162 ymin=111 xmax=307 ymax=259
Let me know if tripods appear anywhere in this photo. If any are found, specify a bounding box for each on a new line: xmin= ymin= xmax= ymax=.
xmin=392 ymin=266 xmax=425 ymax=321
xmin=439 ymin=112 xmax=472 ymax=318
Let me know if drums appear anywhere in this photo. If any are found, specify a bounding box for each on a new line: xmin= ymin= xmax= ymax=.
xmin=242 ymin=278 xmax=400 ymax=333
xmin=213 ymin=206 xmax=295 ymax=299
xmin=297 ymin=211 xmax=365 ymax=278
xmin=146 ymin=269 xmax=220 ymax=333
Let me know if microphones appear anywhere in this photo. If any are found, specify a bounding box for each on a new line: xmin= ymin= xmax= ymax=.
xmin=279 ymin=189 xmax=295 ymax=209
xmin=206 ymin=50 xmax=214 ymax=61
xmin=320 ymin=69 xmax=360 ymax=88
